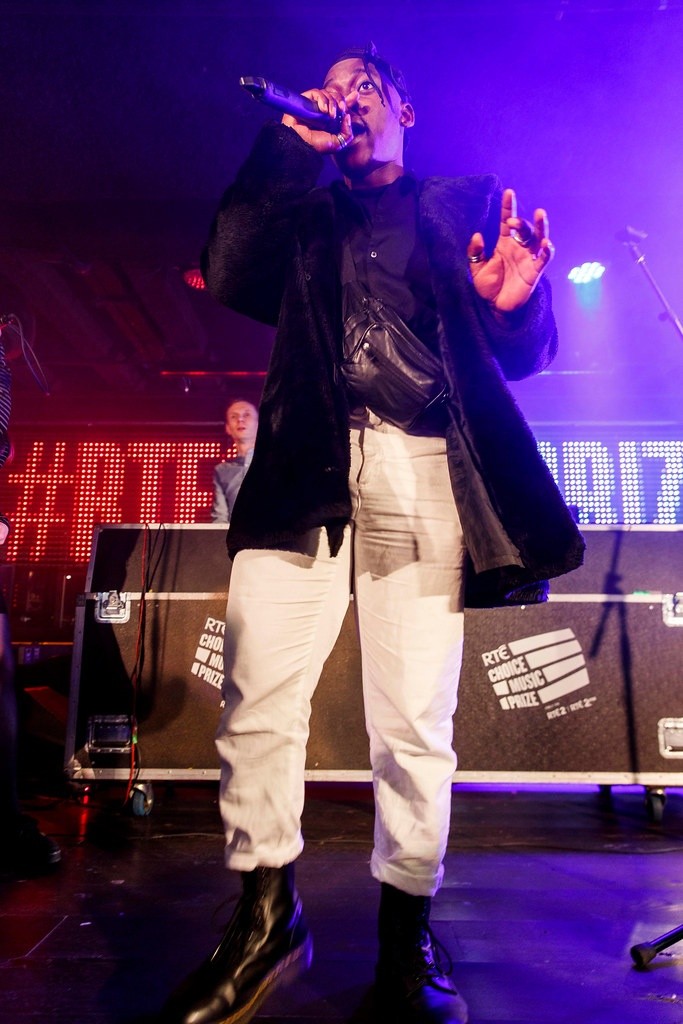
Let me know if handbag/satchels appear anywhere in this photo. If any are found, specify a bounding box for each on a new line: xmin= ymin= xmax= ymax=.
xmin=342 ymin=294 xmax=451 ymax=437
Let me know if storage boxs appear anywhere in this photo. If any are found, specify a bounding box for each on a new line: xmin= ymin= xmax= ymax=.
xmin=65 ymin=525 xmax=683 ymax=819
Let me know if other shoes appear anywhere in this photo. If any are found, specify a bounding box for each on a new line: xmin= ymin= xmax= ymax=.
xmin=2 ymin=811 xmax=65 ymax=876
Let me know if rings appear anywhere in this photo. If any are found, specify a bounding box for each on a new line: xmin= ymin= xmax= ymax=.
xmin=466 ymin=252 xmax=489 ymax=264
xmin=337 ymin=134 xmax=348 ymax=148
xmin=514 ymin=235 xmax=535 ymax=248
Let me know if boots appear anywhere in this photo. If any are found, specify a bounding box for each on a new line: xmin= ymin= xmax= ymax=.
xmin=374 ymin=883 xmax=467 ymax=1024
xmin=165 ymin=861 xmax=313 ymax=1024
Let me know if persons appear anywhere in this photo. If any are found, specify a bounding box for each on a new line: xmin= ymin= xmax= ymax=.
xmin=210 ymin=399 xmax=268 ymax=532
xmin=0 ymin=316 xmax=12 ymax=547
xmin=180 ymin=39 xmax=586 ymax=1024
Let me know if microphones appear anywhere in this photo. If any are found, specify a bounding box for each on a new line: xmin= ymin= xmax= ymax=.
xmin=238 ymin=75 xmax=346 ymax=137
